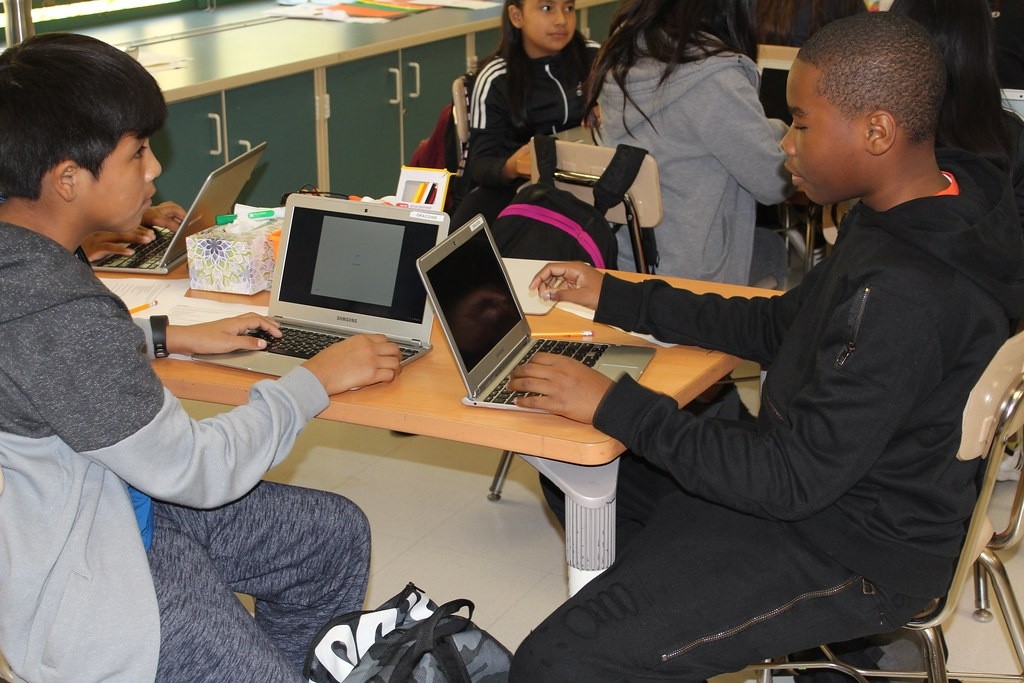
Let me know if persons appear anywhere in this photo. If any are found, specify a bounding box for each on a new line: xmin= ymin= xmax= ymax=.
xmin=597 ymin=0 xmax=799 ymax=291
xmin=1 ymin=34 xmax=404 ymax=683
xmin=79 ymin=200 xmax=186 ymax=264
xmin=510 ymin=12 xmax=1024 ymax=682
xmin=445 ymin=0 xmax=606 ymax=241
xmin=887 ymin=1 xmax=1024 ymax=217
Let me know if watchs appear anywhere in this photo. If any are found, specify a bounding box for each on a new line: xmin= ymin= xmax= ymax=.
xmin=149 ymin=315 xmax=170 ymax=360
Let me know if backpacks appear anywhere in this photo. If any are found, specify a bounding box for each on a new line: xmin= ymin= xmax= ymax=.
xmin=493 ymin=134 xmax=659 ymax=271
xmin=301 ymin=582 xmax=514 ymax=683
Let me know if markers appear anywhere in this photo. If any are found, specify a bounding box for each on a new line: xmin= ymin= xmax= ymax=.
xmin=215 ymin=210 xmax=275 ymax=224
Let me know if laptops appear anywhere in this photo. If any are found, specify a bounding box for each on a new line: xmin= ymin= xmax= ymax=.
xmin=756 ymin=59 xmax=794 ymax=128
xmin=999 ymin=88 xmax=1024 ymax=122
xmin=91 ymin=141 xmax=268 ymax=274
xmin=417 ymin=213 xmax=657 ymax=414
xmin=190 ymin=193 xmax=451 ymax=392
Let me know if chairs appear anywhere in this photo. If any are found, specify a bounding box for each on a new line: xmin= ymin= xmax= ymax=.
xmin=489 ymin=132 xmax=664 ymax=502
xmin=759 ymin=330 xmax=1024 ymax=683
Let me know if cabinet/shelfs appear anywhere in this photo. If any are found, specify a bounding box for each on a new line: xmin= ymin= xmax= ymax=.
xmin=0 ymin=0 xmax=626 ymax=208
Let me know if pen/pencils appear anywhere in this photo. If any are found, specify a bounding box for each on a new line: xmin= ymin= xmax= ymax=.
xmin=412 ymin=182 xmax=439 ymax=205
xmin=530 ymin=331 xmax=595 ymax=338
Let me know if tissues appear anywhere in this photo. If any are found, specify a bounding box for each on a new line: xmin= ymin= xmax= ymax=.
xmin=185 ymin=202 xmax=284 ymax=296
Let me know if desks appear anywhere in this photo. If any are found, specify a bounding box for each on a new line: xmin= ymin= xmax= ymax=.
xmin=97 ymin=254 xmax=787 ymax=598
